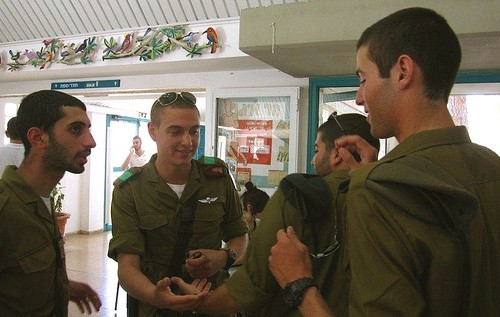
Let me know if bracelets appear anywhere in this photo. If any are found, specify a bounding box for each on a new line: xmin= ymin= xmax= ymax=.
xmin=220 ymin=247 xmax=237 ymax=271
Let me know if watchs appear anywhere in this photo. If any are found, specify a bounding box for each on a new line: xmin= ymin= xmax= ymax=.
xmin=283 ymin=277 xmax=319 ymax=309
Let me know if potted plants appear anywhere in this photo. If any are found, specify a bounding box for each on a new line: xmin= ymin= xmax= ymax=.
xmin=51 ymin=181 xmax=71 ymax=236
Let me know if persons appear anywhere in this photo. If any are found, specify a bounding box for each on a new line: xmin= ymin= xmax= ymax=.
xmin=239 ymin=180 xmax=270 ymax=239
xmin=0 ymin=117 xmax=24 ymax=178
xmin=170 ymin=114 xmax=380 ymax=317
xmin=121 ymin=135 xmax=151 ymax=172
xmin=108 ymin=93 xmax=250 ymax=317
xmin=0 ymin=90 xmax=102 ymax=317
xmin=268 ymin=6 xmax=500 ymax=317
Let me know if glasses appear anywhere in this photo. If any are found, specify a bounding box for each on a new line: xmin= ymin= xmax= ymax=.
xmin=280 ymin=189 xmax=341 ymax=260
xmin=153 ymin=92 xmax=196 ymax=107
xmin=328 ymin=111 xmax=345 ymax=131
